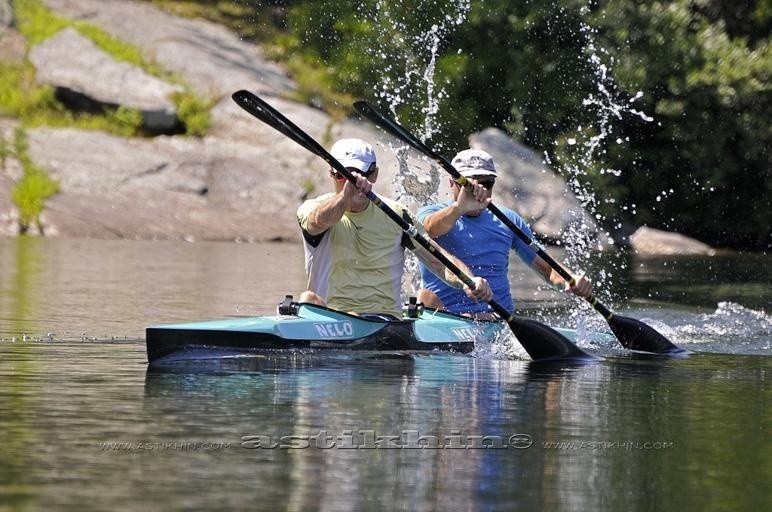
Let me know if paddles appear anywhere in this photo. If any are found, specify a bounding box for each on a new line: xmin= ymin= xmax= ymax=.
xmin=232 ymin=89 xmax=589 ymax=360
xmin=354 ymin=97 xmax=680 ymax=355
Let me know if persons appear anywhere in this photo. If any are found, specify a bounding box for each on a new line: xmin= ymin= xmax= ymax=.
xmin=416 ymin=148 xmax=593 ymax=321
xmin=297 ymin=137 xmax=493 ymax=322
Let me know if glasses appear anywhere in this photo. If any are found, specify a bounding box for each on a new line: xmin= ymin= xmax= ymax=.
xmin=450 ymin=177 xmax=495 ymax=193
xmin=329 ymin=163 xmax=375 ymax=183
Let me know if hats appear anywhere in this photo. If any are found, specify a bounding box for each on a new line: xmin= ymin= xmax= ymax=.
xmin=449 ymin=149 xmax=497 ymax=182
xmin=327 ymin=139 xmax=376 ymax=173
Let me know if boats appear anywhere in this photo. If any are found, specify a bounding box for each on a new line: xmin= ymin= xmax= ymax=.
xmin=145 ymin=295 xmax=619 ymax=361
